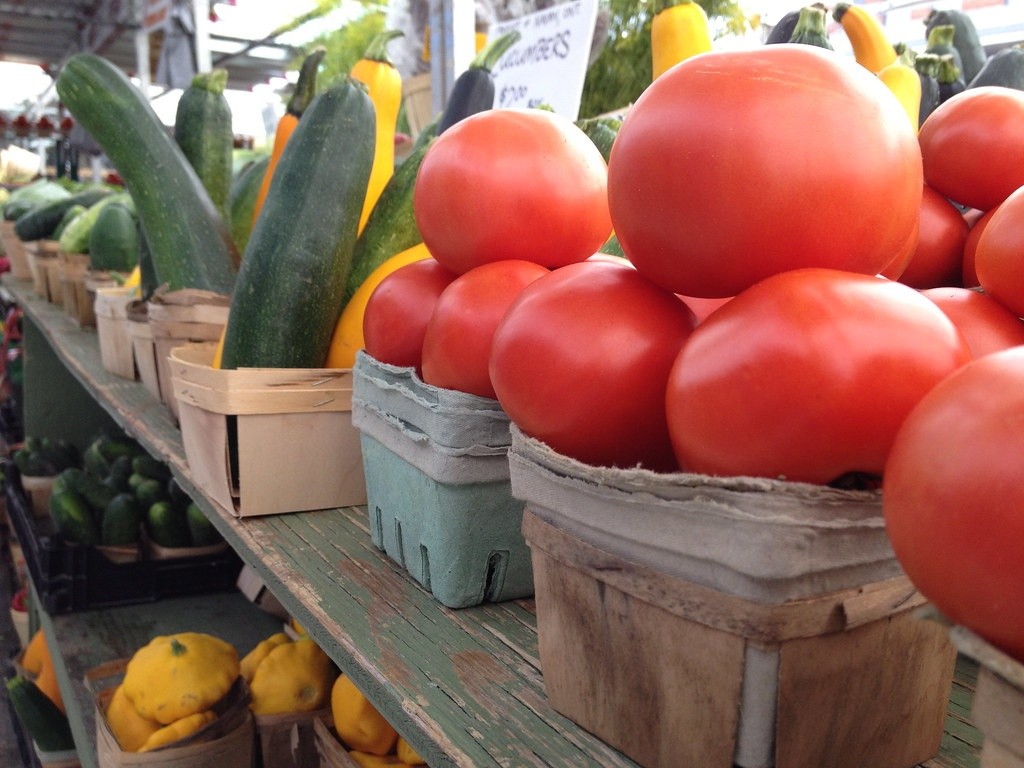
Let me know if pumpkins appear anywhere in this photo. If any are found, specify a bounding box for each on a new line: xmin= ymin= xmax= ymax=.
xmin=21 ymin=615 xmax=425 ymax=768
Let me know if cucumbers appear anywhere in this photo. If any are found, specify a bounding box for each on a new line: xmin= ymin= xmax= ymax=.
xmin=14 ymin=432 xmax=222 ymax=550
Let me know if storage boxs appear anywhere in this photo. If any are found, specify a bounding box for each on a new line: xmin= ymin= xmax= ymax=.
xmin=351 ymin=351 xmax=534 ymax=608
xmin=507 ymin=421 xmax=958 ymax=768
xmin=0 ymin=473 xmax=368 ymax=768
xmin=0 ymin=223 xmax=367 ymax=517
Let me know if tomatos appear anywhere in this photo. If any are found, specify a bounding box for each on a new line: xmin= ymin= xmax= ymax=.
xmin=362 ymin=42 xmax=1024 ymax=660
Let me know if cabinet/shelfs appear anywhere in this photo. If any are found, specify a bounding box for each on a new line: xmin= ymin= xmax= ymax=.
xmin=0 ymin=272 xmax=984 ymax=768
